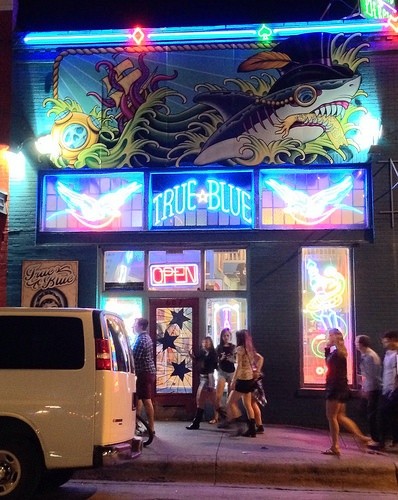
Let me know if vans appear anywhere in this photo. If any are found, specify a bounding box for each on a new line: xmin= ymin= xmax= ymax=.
xmin=0 ymin=306 xmax=145 ymax=500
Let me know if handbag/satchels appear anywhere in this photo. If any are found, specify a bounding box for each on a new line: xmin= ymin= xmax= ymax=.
xmin=255 ymin=380 xmax=265 ymax=395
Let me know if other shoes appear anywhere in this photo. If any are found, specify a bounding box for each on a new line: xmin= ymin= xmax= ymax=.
xmin=321 ymin=450 xmax=341 ymax=455
xmin=186 ymin=423 xmax=199 ymax=429
xmin=243 ymin=430 xmax=257 ymax=437
xmin=218 ymin=422 xmax=229 ymax=427
xmin=209 ymin=417 xmax=218 ymax=424
xmin=255 ymin=428 xmax=265 ymax=434
xmin=136 ymin=429 xmax=155 ymax=436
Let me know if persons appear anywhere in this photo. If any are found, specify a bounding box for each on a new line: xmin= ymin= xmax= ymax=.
xmin=186 ymin=327 xmax=267 ymax=438
xmin=354 ymin=332 xmax=398 ymax=450
xmin=132 ymin=319 xmax=158 ymax=436
xmin=321 ymin=328 xmax=372 ymax=455
